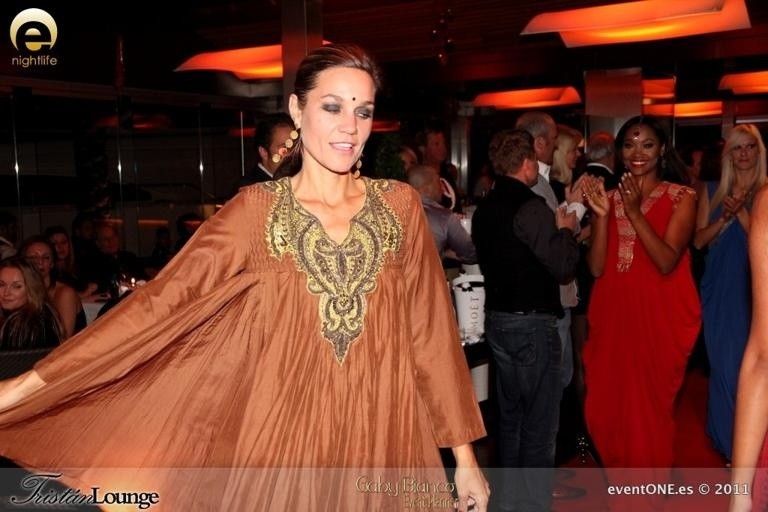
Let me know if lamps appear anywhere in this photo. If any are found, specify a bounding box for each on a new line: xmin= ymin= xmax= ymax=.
xmin=516 ymin=2 xmax=753 ymax=52
xmin=469 ymin=69 xmax=768 ymax=120
xmin=177 ymin=40 xmax=334 ymax=84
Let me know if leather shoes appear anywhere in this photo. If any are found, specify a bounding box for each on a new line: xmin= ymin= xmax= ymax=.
xmin=549 ymin=483 xmax=586 ymax=499
xmin=552 ymin=466 xmax=577 ymax=481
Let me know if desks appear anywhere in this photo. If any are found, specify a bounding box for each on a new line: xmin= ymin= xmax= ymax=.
xmin=79 ymin=288 xmax=114 ymax=325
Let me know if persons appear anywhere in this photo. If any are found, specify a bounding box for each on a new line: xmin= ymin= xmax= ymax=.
xmin=1 ymin=110 xmax=768 ymax=511
xmin=2 ymin=42 xmax=491 ymax=512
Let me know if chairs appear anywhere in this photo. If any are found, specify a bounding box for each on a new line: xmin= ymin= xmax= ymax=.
xmin=0 ymin=343 xmax=60 ymax=382
